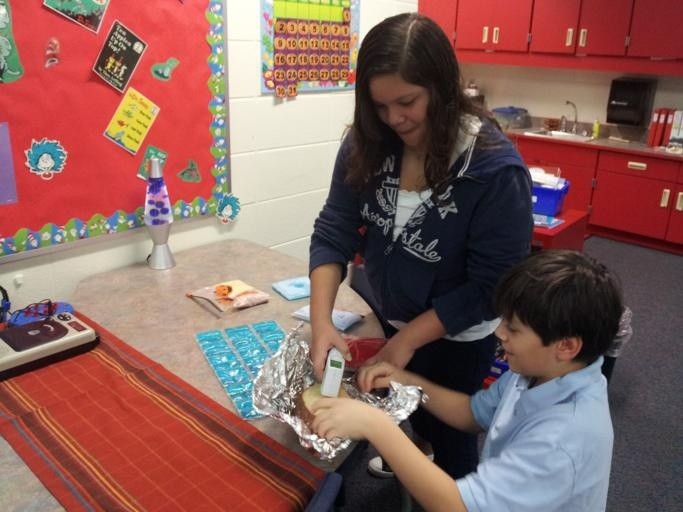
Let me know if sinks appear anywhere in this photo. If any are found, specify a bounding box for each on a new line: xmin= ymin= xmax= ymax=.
xmin=523 ymin=129 xmax=595 ymax=142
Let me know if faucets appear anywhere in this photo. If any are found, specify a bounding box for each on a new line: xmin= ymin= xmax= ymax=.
xmin=565 ymin=100 xmax=578 ymax=134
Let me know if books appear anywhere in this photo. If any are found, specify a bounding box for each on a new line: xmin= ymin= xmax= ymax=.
xmin=647 ymin=108 xmax=683 ymax=155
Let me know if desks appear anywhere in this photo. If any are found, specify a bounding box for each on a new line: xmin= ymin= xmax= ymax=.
xmin=1 ymin=240 xmax=384 ymax=512
xmin=532 ymin=208 xmax=590 ymax=257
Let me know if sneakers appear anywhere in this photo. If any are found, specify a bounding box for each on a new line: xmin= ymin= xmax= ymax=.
xmin=367 ymin=453 xmax=435 ymax=478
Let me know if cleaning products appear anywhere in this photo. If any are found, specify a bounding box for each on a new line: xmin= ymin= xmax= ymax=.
xmin=593 ymin=117 xmax=600 ymax=137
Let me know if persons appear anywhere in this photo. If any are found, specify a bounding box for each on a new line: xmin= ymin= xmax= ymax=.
xmin=309 ymin=247 xmax=625 ymax=512
xmin=307 ymin=13 xmax=536 ymax=479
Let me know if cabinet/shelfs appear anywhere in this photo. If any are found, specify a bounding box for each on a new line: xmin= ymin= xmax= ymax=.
xmin=457 ymin=1 xmax=535 ymax=66
xmin=530 ymin=0 xmax=635 ymax=74
xmin=514 ymin=136 xmax=601 ymax=214
xmin=587 ymin=149 xmax=683 ymax=258
xmin=418 ymin=0 xmax=458 ymax=62
xmin=628 ymin=1 xmax=682 ymax=74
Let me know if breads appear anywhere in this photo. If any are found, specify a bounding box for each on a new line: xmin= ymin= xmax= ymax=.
xmin=296 ymin=382 xmax=352 ymax=420
